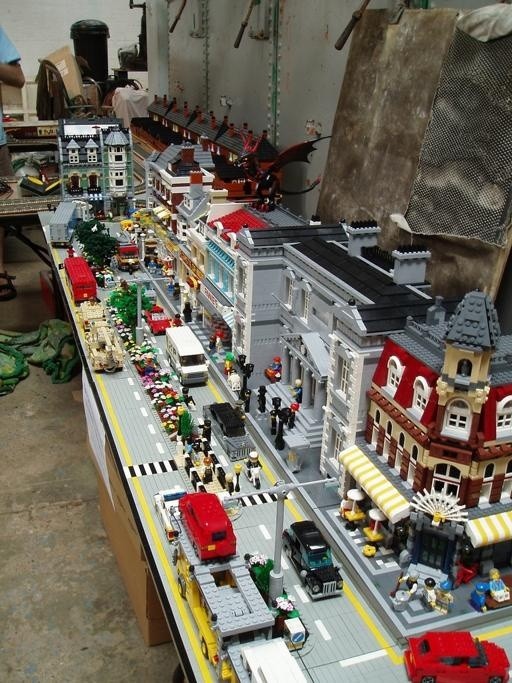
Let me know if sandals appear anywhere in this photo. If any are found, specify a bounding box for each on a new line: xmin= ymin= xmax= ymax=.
xmin=0 ymin=270 xmax=17 ymax=302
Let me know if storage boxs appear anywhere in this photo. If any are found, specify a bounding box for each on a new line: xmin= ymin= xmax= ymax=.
xmin=83 ymin=384 xmax=174 ymax=649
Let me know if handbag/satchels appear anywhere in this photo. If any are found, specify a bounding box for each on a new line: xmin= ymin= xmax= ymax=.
xmin=34 ymin=59 xmax=70 ymax=121
